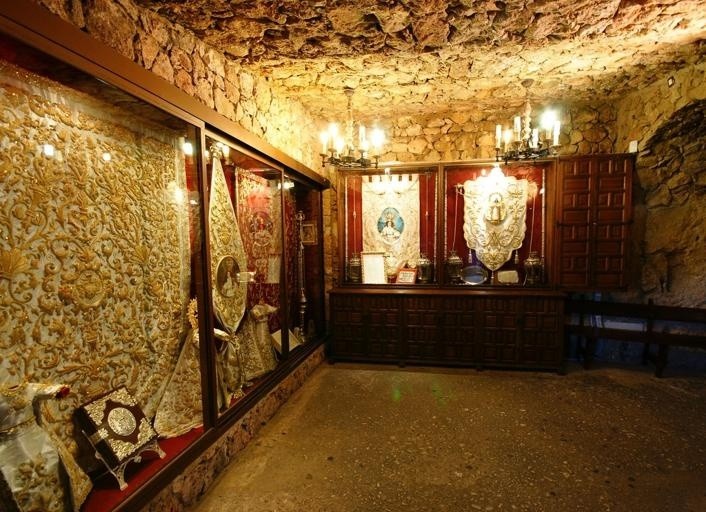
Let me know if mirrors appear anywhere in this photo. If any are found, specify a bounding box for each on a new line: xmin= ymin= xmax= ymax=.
xmin=456 ymin=266 xmax=490 ymax=285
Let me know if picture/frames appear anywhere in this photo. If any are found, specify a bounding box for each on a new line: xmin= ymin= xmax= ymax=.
xmin=360 ymin=251 xmax=418 ymax=285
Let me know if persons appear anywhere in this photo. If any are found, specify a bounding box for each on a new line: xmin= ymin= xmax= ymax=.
xmin=221 ymin=271 xmax=238 ymax=297
xmin=380 ymin=221 xmax=401 ymax=243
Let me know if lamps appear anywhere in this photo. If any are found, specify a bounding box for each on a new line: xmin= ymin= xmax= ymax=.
xmin=317 ymin=87 xmax=386 ymax=170
xmin=495 ymin=97 xmax=562 ymax=162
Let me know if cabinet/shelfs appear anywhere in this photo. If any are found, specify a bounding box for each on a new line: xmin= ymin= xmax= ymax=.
xmin=325 ymin=286 xmax=569 ymax=375
xmin=545 ymin=156 xmax=633 ymax=291
